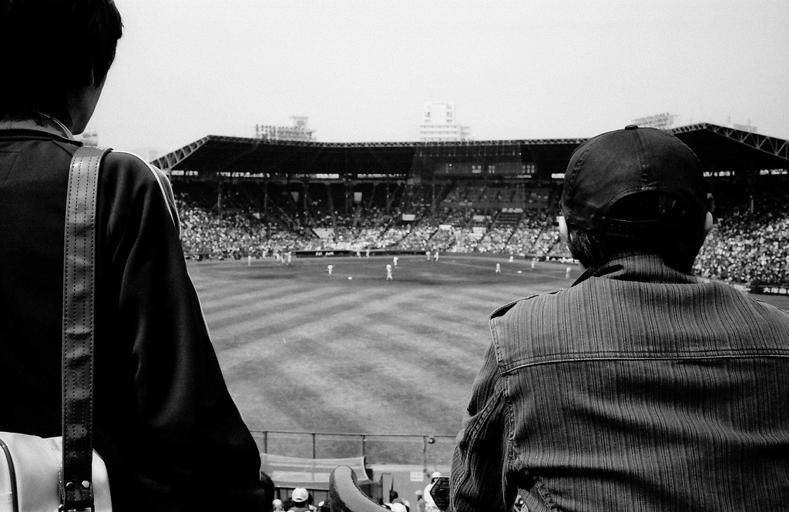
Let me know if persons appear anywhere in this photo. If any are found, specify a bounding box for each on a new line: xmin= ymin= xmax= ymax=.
xmin=1 ymin=0 xmax=279 ymax=512
xmin=434 ymin=124 xmax=789 ymax=512
xmin=691 ymin=201 xmax=789 ymax=286
xmin=270 ymin=470 xmax=444 ymax=511
xmin=165 ymin=181 xmax=574 ymax=280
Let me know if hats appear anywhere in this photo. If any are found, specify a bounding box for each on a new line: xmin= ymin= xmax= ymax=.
xmin=432 ymin=472 xmax=442 ymax=478
xmin=415 ymin=490 xmax=423 ymax=495
xmin=559 ymin=124 xmax=706 ymax=239
xmin=291 ymin=487 xmax=310 ymax=502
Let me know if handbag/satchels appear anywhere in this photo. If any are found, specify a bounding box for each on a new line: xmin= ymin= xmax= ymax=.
xmin=0 ymin=429 xmax=114 ymax=512
